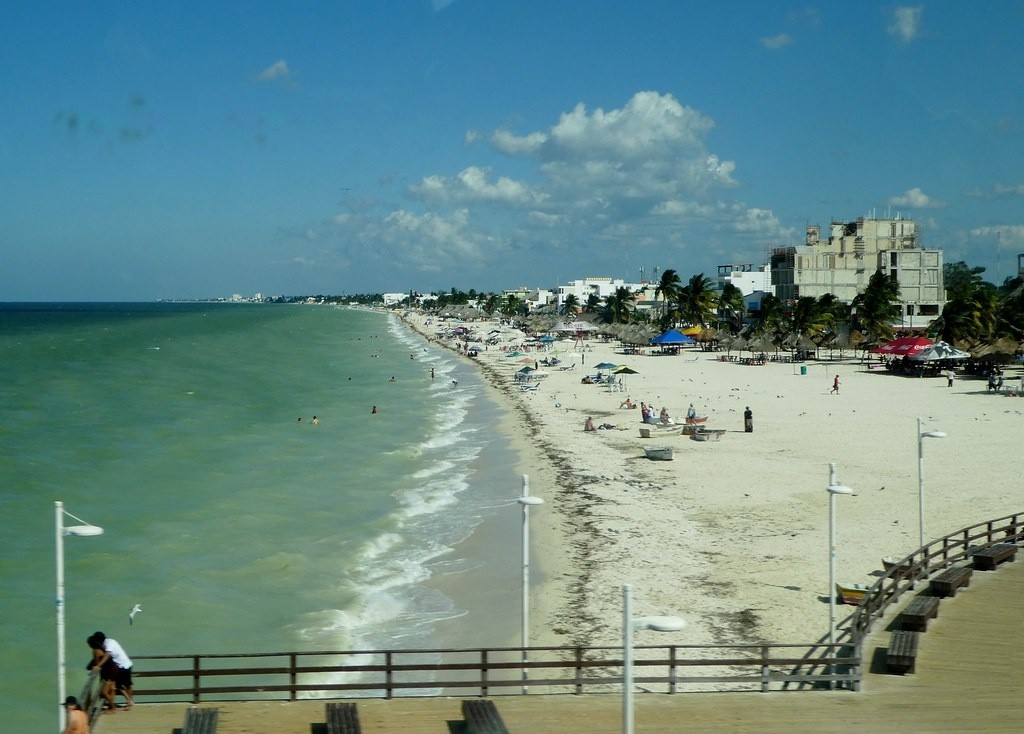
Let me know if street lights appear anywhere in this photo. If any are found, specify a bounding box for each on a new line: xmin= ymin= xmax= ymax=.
xmin=622 ymin=584 xmax=687 ymax=734
xmin=55 ymin=501 xmax=103 ymax=734
xmin=917 ymin=417 xmax=946 ymax=572
xmin=826 ymin=462 xmax=856 ymax=691
xmin=516 ymin=475 xmax=544 ymax=694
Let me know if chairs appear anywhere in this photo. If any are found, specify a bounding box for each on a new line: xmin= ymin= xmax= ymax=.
xmin=560 ymin=364 xmax=576 ymax=371
xmin=520 ymin=383 xmax=540 ymax=392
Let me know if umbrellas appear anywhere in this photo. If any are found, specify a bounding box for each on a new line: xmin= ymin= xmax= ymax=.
xmin=718 ymin=334 xmax=816 ymax=359
xmin=594 ymin=363 xmax=639 ymax=385
xmin=469 ymin=346 xmax=481 ymax=351
xmin=506 ymin=351 xmax=535 ymax=363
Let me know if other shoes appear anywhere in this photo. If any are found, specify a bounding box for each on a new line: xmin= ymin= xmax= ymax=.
xmin=100 ymin=706 xmax=117 ymax=715
xmin=124 ymin=700 xmax=134 ymax=710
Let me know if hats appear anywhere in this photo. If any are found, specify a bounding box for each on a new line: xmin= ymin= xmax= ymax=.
xmin=62 ymin=696 xmax=77 ymax=706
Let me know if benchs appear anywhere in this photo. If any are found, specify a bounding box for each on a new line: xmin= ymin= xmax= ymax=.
xmin=930 ymin=567 xmax=973 ymax=597
xmin=900 ymin=595 xmax=940 ymax=632
xmin=462 ymin=699 xmax=509 ymax=734
xmin=970 ymin=544 xmax=1018 ymax=570
xmin=181 ymin=706 xmax=219 ymax=734
xmin=885 ymin=629 xmax=919 ymax=674
xmin=326 ymin=702 xmax=361 ymax=734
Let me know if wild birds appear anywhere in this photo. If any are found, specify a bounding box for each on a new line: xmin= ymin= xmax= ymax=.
xmin=129 ymin=605 xmax=143 ymax=624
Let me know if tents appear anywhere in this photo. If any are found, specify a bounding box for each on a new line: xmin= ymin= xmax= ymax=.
xmin=651 ymin=326 xmax=702 ymax=353
xmin=870 ymin=337 xmax=971 ymax=360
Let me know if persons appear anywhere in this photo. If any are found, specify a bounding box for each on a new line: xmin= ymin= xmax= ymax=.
xmin=456 ymin=342 xmax=477 ymax=357
xmin=373 ymin=406 xmax=376 ymax=414
xmin=582 ymin=372 xmax=601 ymax=383
xmin=585 ymin=417 xmax=596 ymax=431
xmin=687 ymin=403 xmax=696 ymax=417
xmin=619 ymin=399 xmax=636 ymax=409
xmin=989 ymin=374 xmax=1003 ymax=390
xmin=390 ymin=377 xmax=396 ymax=382
xmin=744 ymin=406 xmax=753 ymax=432
xmin=947 ymin=370 xmax=955 ymax=387
xmin=431 ymin=368 xmax=434 ymax=377
xmin=62 ymin=695 xmax=90 ymax=734
xmin=312 ymin=416 xmax=318 ymax=424
xmin=87 ymin=631 xmax=134 ymax=715
xmin=830 ymin=374 xmax=841 ymax=394
xmin=660 ymin=407 xmax=671 ymax=423
xmin=641 ymin=402 xmax=654 ymax=418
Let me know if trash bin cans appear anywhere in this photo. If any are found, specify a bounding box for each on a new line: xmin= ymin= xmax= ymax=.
xmin=800 ymin=366 xmax=807 ymax=375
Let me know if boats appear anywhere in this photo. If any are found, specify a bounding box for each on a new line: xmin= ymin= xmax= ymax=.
xmin=646 ymin=417 xmax=674 ymax=425
xmin=639 ymin=424 xmax=684 ymax=438
xmin=644 ymin=446 xmax=674 ymax=460
xmin=695 ymin=427 xmax=726 ymax=442
xmin=675 ymin=416 xmax=708 ymax=425
xmin=657 ymin=423 xmax=705 ymax=435
xmin=882 ymin=555 xmax=934 ymax=579
xmin=836 ymin=581 xmax=903 ymax=607
